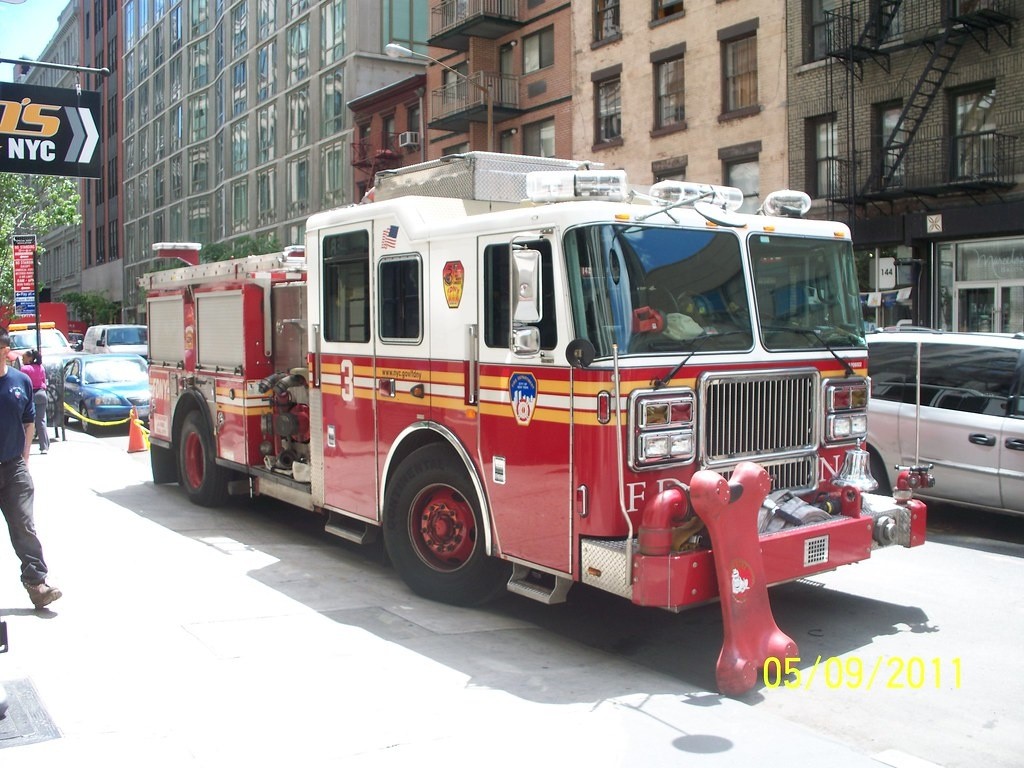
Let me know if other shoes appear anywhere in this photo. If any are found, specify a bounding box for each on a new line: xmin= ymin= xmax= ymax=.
xmin=41 ymin=448 xmax=48 ymax=453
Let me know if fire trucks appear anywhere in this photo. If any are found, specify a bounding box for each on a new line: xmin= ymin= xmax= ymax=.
xmin=135 ymin=151 xmax=936 ymax=696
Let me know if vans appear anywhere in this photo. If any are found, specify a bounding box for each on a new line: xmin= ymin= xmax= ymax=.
xmin=865 ymin=327 xmax=1024 ymax=527
xmin=83 ymin=325 xmax=148 ymax=361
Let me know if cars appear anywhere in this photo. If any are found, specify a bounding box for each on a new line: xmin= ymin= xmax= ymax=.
xmin=6 ymin=322 xmax=83 ymax=424
xmin=62 ymin=353 xmax=151 ymax=434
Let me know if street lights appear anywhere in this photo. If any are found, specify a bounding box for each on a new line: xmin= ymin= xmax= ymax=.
xmin=386 ymin=42 xmax=492 ymax=153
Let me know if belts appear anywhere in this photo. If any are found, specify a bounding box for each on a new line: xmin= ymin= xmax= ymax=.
xmin=0 ymin=456 xmax=21 ymax=468
xmin=33 ymin=388 xmax=44 ymax=393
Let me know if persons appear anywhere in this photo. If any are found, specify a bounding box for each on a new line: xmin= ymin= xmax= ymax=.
xmin=0 ymin=326 xmax=62 ymax=609
xmin=20 ymin=350 xmax=50 ymax=454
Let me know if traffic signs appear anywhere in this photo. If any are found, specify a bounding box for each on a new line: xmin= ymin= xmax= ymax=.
xmin=0 ymin=83 xmax=104 ymax=179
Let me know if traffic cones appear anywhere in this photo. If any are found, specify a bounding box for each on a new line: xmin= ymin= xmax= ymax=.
xmin=126 ymin=405 xmax=147 ymax=453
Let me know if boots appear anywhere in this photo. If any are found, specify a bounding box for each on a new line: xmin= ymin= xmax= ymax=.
xmin=21 ymin=577 xmax=62 ymax=608
